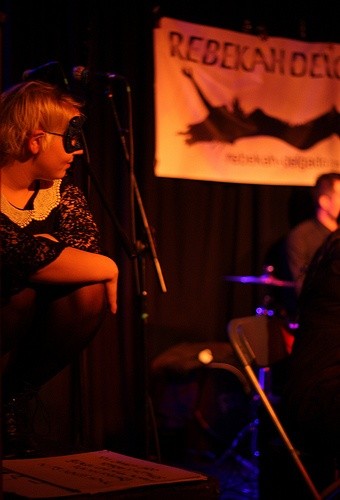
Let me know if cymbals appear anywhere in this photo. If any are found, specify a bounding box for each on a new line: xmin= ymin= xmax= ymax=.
xmin=223 ymin=275 xmax=301 ymax=289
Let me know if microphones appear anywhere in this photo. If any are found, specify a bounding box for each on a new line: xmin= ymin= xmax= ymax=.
xmin=73 ymin=65 xmax=125 ymax=81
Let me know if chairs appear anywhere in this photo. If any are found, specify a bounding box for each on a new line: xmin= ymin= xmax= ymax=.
xmin=225 ymin=316 xmax=340 ymax=500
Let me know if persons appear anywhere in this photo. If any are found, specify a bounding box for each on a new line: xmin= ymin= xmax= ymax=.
xmin=286 ymin=171 xmax=340 ymax=298
xmin=0 ymin=76 xmax=120 ymax=461
xmin=273 ymin=219 xmax=340 ymax=499
